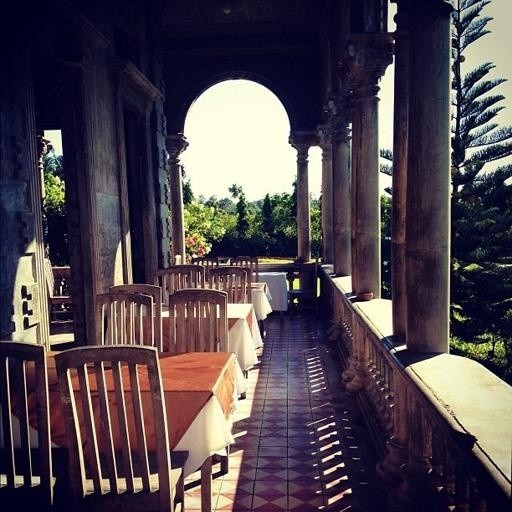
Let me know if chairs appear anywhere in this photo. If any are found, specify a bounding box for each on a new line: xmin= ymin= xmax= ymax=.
xmin=107 ymin=285 xmax=163 ymax=351
xmin=0 ymin=339 xmax=61 ymax=512
xmin=166 ymin=265 xmax=205 ymax=289
xmin=287 ymin=258 xmax=320 ymax=319
xmin=235 ymin=256 xmax=259 ymax=282
xmin=92 ymin=284 xmax=165 ymax=353
xmin=52 ymin=343 xmax=190 ymax=512
xmin=154 ymin=264 xmax=207 ymax=307
xmin=190 ymin=256 xmax=219 ymax=268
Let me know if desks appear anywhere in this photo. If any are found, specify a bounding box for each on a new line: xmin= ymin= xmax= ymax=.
xmin=211 ymin=271 xmax=288 ymax=312
